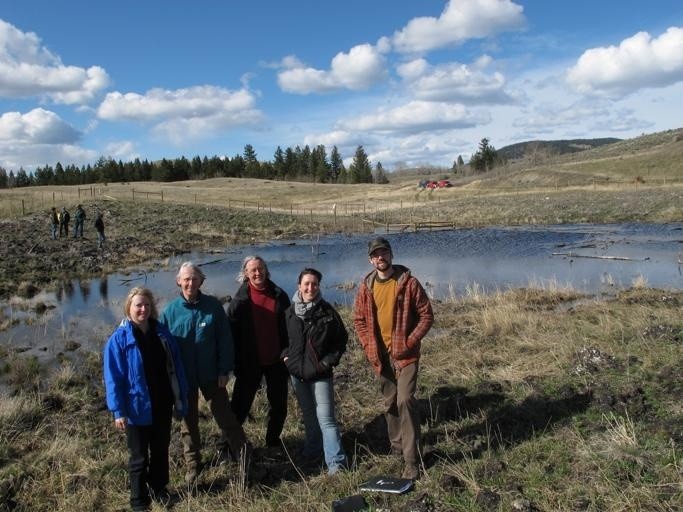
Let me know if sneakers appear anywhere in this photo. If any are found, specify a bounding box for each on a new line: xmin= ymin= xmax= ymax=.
xmin=402 ymin=464 xmax=421 ymax=481
xmin=185 ymin=461 xmax=203 ymax=482
xmin=148 ymin=487 xmax=175 ymax=508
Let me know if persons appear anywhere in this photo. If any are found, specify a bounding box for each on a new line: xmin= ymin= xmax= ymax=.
xmin=276 ymin=266 xmax=349 ymax=476
xmin=354 ymin=235 xmax=436 ymax=480
xmin=69 ymin=203 xmax=86 ymax=239
xmin=93 ymin=213 xmax=105 ymax=250
xmin=48 ymin=206 xmax=59 ymax=241
xmin=58 ymin=206 xmax=70 ymax=238
xmin=158 ymin=260 xmax=271 ymax=487
xmin=54 ymin=276 xmax=111 ymax=308
xmin=222 ymin=254 xmax=292 ymax=448
xmin=102 ymin=285 xmax=190 ymax=512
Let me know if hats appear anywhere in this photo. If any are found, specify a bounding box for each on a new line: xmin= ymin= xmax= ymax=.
xmin=368 ymin=236 xmax=391 ymax=255
